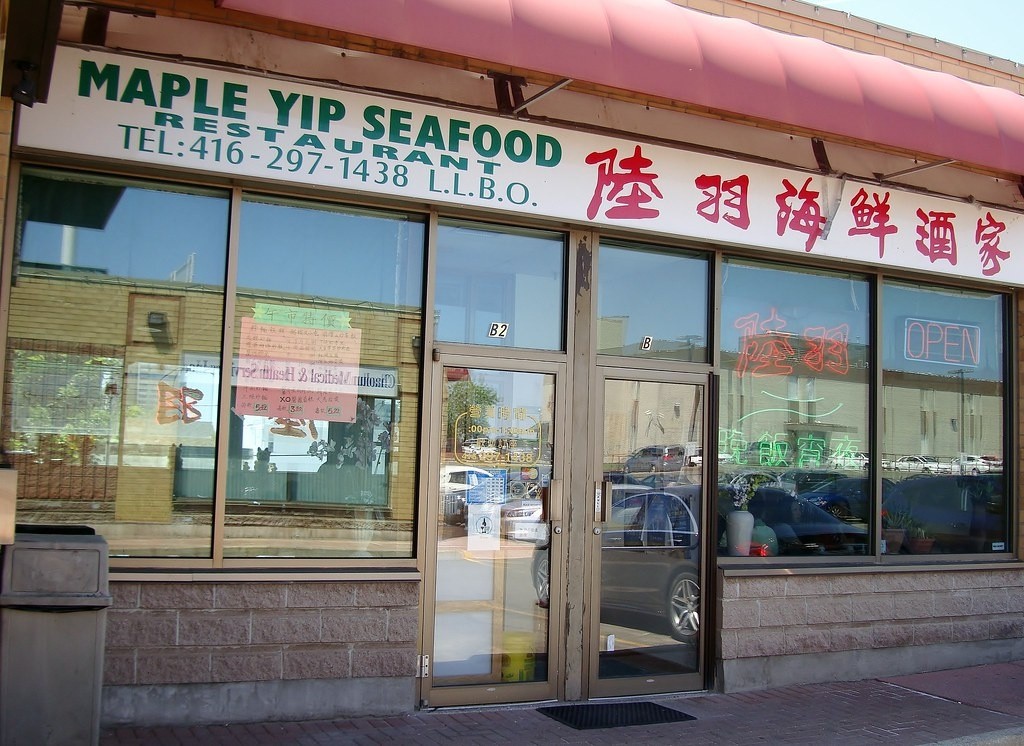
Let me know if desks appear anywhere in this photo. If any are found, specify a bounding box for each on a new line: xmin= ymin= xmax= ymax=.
xmin=433 ymin=526 xmax=536 ymax=687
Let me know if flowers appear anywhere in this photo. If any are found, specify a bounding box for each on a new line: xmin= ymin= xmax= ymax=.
xmin=881 ymin=510 xmax=906 ymax=529
xmin=305 ymin=396 xmax=397 ymax=509
xmin=725 ymin=473 xmax=768 ymax=512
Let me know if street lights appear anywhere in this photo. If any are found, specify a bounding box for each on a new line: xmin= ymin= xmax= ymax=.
xmin=947 ymin=368 xmax=975 ymax=464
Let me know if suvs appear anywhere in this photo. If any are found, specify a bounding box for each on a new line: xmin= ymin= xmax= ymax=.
xmin=888 ymin=469 xmax=1006 ymax=553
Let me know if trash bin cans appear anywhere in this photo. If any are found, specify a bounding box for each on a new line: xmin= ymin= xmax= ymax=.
xmin=0 ymin=534 xmax=114 ymax=746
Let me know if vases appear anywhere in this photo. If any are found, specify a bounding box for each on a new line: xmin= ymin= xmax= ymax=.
xmin=881 ymin=528 xmax=906 ymax=556
xmin=352 ymin=506 xmax=375 ymax=558
xmin=725 ymin=511 xmax=756 ymax=558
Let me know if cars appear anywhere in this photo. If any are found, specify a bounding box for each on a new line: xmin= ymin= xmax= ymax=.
xmin=689 ymin=441 xmax=1002 ymax=475
xmin=716 ymin=469 xmax=895 ymax=520
xmin=531 ymin=484 xmax=869 ymax=642
xmin=622 ymin=444 xmax=685 ymax=474
xmin=440 ymin=465 xmax=653 ymax=541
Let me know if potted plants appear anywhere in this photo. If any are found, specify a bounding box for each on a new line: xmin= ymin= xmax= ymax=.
xmin=903 ymin=514 xmax=935 ymax=556
